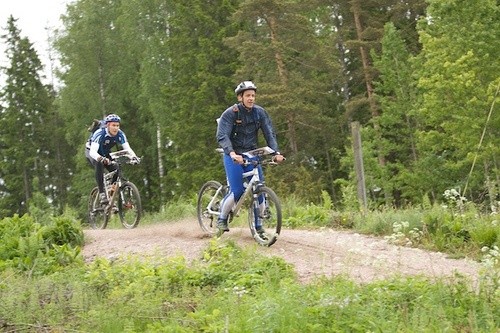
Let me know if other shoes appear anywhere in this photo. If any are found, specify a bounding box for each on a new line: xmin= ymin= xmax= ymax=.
xmin=111 ymin=205 xmax=119 ymax=214
xmin=256 ymin=229 xmax=268 ymax=240
xmin=216 ymin=218 xmax=229 ymax=231
xmin=99 ymin=193 xmax=109 ymax=204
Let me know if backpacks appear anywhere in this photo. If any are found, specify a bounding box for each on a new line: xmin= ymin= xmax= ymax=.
xmin=85 ymin=120 xmax=121 ymax=166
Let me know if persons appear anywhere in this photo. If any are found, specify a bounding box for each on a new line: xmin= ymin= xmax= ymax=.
xmin=216 ymin=81 xmax=285 ymax=243
xmin=85 ymin=114 xmax=139 ymax=212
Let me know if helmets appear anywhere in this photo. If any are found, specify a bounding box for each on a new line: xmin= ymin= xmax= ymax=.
xmin=104 ymin=114 xmax=120 ymax=123
xmin=235 ymin=81 xmax=257 ymax=95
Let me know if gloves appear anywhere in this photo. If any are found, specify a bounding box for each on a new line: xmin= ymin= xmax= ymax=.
xmin=98 ymin=156 xmax=110 ymax=165
xmin=133 ymin=156 xmax=140 ymax=164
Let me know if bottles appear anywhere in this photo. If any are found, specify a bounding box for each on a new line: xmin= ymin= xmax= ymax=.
xmin=110 ymin=182 xmax=117 ymax=198
xmin=236 ymin=180 xmax=249 ymax=203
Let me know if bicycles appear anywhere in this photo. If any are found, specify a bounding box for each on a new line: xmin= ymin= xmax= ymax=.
xmin=87 ymin=156 xmax=145 ymax=230
xmin=197 ymin=155 xmax=287 ymax=248
